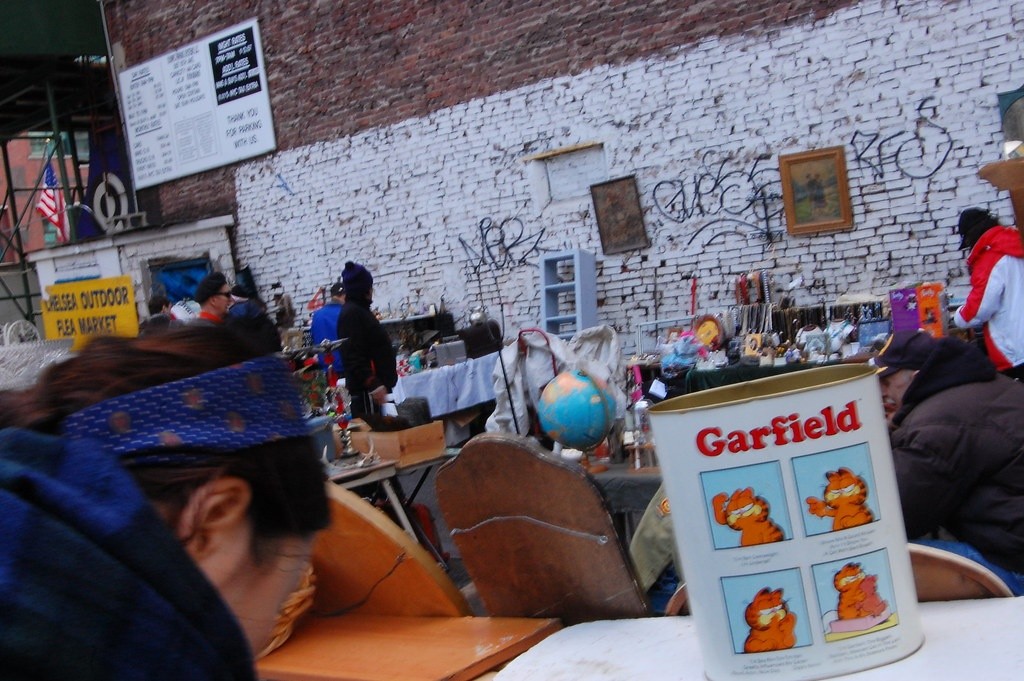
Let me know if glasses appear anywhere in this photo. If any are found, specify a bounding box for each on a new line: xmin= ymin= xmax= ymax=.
xmin=212 ymin=291 xmax=232 ymax=297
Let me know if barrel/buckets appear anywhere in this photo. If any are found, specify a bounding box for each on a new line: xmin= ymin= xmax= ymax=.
xmin=648 ymin=364 xmax=926 ymax=681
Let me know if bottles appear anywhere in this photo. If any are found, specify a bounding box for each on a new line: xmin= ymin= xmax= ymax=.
xmin=775 ymin=357 xmax=786 ymax=366
xmin=760 ymin=356 xmax=772 ymax=366
xmin=408 ymin=354 xmax=421 ymax=370
xmin=607 ymin=419 xmax=626 ymax=464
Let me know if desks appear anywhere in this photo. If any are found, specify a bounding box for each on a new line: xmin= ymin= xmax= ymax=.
xmin=590 ymin=467 xmax=665 ymax=549
xmin=623 ymin=357 xmax=663 ymax=397
xmin=374 ymin=350 xmax=512 ymax=441
xmin=250 ymin=611 xmax=559 ymax=681
xmin=489 ymin=597 xmax=1024 ymax=681
xmin=396 ymin=447 xmax=462 ymax=568
xmin=287 ymin=312 xmax=442 ymax=331
xmin=319 ymin=458 xmax=419 ymax=544
xmin=687 ymin=349 xmax=874 ymax=397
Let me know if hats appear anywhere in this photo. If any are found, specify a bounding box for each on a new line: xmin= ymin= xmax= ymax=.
xmin=331 ymin=283 xmax=344 ymax=294
xmin=195 ymin=272 xmax=226 ymax=303
xmin=343 ymin=262 xmax=373 ymax=297
xmin=867 ymin=327 xmax=934 ymax=377
xmin=958 ymin=207 xmax=999 ymax=250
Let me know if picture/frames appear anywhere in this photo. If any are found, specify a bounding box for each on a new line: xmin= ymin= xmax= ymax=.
xmin=588 ymin=174 xmax=649 ymax=255
xmin=777 ymin=146 xmax=853 ymax=236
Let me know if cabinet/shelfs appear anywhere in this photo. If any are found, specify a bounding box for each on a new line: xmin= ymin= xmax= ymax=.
xmin=538 ymin=246 xmax=596 ymax=339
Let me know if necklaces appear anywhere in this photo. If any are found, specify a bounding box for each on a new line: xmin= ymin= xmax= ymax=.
xmin=762 ymin=270 xmax=771 ymax=303
xmin=827 ymin=320 xmax=850 ymax=341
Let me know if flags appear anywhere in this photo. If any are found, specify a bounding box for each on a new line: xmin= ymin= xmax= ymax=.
xmin=33 ymin=160 xmax=71 ymax=243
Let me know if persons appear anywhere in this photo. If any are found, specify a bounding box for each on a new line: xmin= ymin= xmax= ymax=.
xmin=953 ymin=207 xmax=1024 ymax=388
xmin=751 ymin=339 xmax=757 ymax=350
xmin=310 ymin=260 xmax=408 ymax=509
xmin=871 ymin=329 xmax=1024 ymax=580
xmin=138 ymin=272 xmax=265 ymax=341
xmin=0 ymin=313 xmax=332 ymax=681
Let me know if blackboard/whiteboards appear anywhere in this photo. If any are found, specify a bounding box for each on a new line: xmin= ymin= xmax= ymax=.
xmin=117 ymin=16 xmax=276 ymax=191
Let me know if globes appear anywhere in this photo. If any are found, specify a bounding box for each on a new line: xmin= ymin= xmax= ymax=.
xmin=538 ymin=370 xmax=616 ymax=473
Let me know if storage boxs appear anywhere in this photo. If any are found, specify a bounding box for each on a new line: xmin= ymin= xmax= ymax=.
xmin=343 ymin=418 xmax=444 ymax=468
